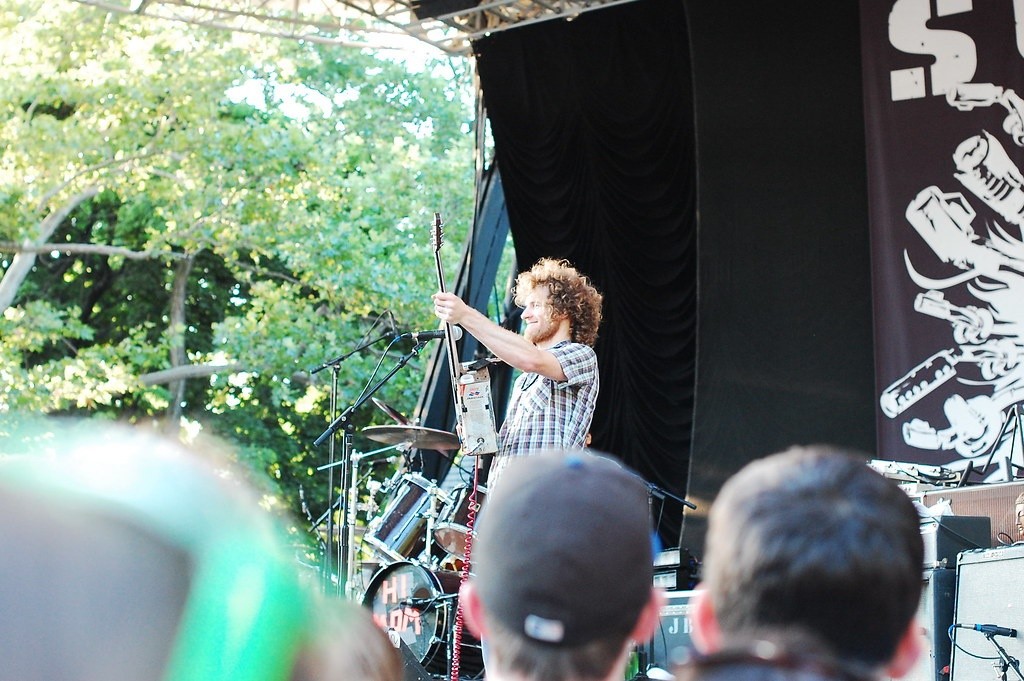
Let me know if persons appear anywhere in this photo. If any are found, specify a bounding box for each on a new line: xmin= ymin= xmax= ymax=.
xmin=0 ymin=409 xmax=318 ymax=681
xmin=431 ymin=253 xmax=604 ymax=500
xmin=457 ymin=449 xmax=660 ymax=681
xmin=291 ymin=593 xmax=405 ymax=681
xmin=668 ymin=444 xmax=931 ymax=681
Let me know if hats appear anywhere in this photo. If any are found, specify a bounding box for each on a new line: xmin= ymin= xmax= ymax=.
xmin=471 ymin=448 xmax=655 ymax=648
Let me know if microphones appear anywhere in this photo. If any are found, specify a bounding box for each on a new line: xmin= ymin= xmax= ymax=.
xmin=368 ymin=455 xmax=397 ymax=465
xmin=389 ymin=310 xmax=400 ymax=341
xmin=953 ymin=623 xmax=1017 ymax=638
xmin=401 ymin=325 xmax=462 ymax=340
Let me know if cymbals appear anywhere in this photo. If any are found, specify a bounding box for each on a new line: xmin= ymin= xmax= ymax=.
xmin=360 ymin=425 xmax=461 ymax=450
xmin=370 ymin=396 xmax=450 ymax=457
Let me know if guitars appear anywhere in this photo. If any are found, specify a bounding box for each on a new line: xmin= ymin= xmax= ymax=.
xmin=431 ymin=212 xmax=503 ymax=456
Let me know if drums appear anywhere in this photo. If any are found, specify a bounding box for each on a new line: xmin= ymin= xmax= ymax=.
xmin=357 ymin=559 xmax=485 ymax=678
xmin=361 ymin=472 xmax=450 ymax=563
xmin=431 ymin=482 xmax=487 ymax=559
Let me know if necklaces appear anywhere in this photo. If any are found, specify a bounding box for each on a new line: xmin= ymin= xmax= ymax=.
xmin=519 ymin=372 xmax=539 ymax=391
